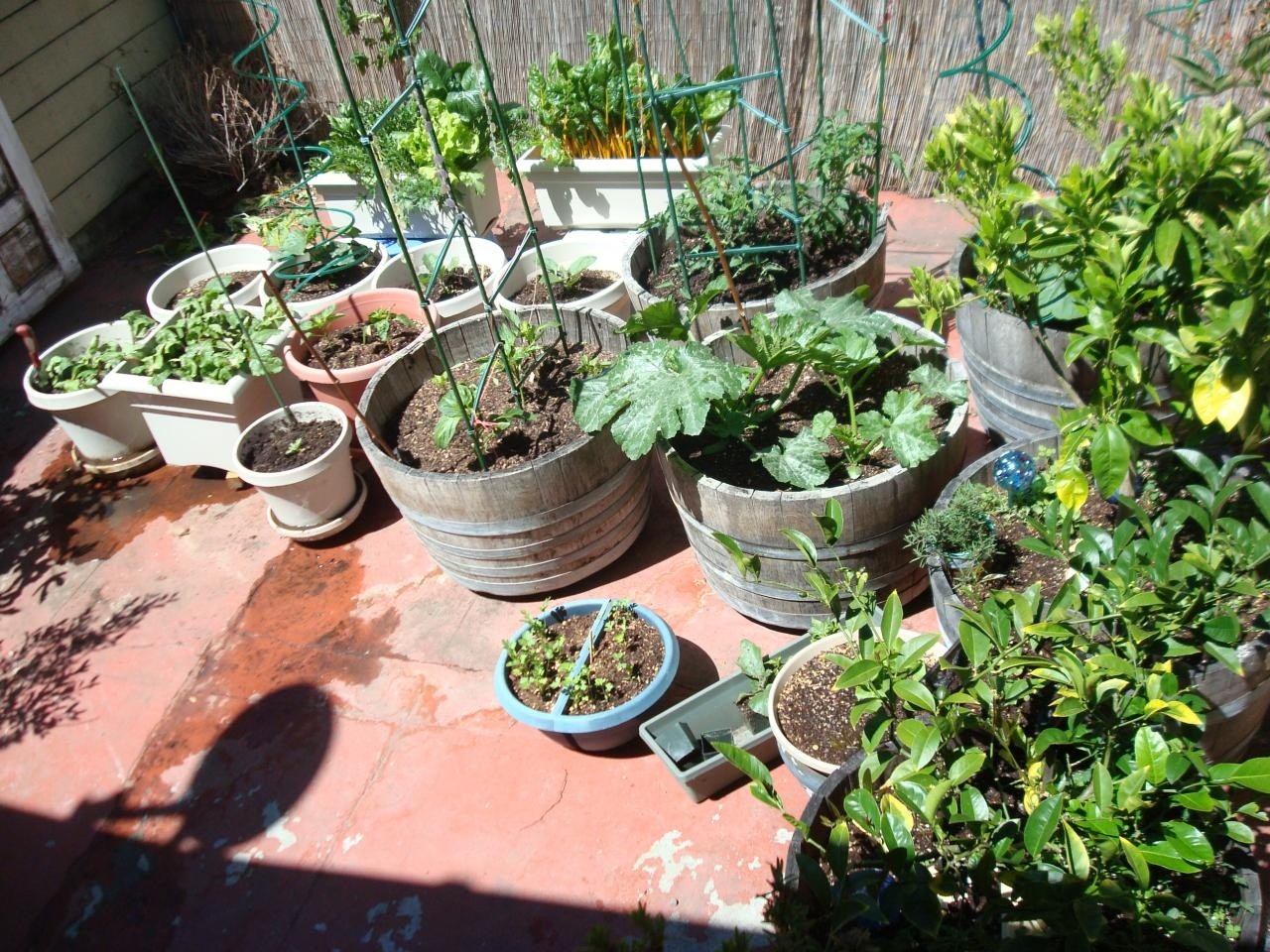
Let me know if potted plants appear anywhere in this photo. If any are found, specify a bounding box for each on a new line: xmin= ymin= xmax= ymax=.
xmin=24 ymin=1 xmax=1270 ymax=952
